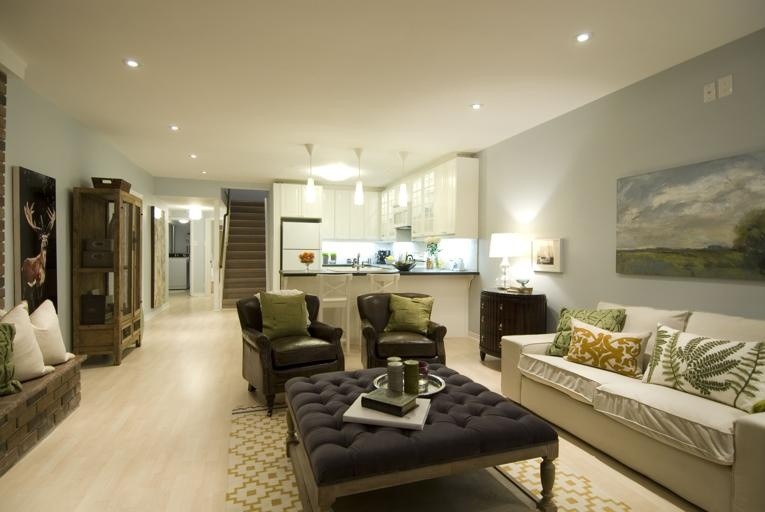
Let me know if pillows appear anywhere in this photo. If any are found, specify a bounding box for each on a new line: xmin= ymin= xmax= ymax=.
xmin=2 ymin=302 xmax=54 ymax=385
xmin=0 ymin=323 xmax=22 ymax=395
xmin=643 ymin=322 xmax=765 ymax=415
xmin=29 ymin=299 xmax=75 ymax=366
xmin=385 ymin=294 xmax=435 ymax=335
xmin=563 ymin=317 xmax=651 ymax=380
xmin=260 ymin=291 xmax=312 ymax=340
xmin=546 ymin=308 xmax=626 ymax=359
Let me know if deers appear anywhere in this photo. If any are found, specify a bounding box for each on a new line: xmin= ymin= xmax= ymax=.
xmin=21 ymin=201 xmax=56 ymax=310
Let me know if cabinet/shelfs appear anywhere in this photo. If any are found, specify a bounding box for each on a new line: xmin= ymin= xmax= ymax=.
xmin=71 ymin=188 xmax=144 ymax=366
xmin=480 ymin=288 xmax=547 ymax=362
xmin=274 ymin=156 xmax=478 ymax=242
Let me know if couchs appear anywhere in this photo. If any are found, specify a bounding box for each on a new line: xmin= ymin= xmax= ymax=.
xmin=502 ymin=302 xmax=765 ymax=512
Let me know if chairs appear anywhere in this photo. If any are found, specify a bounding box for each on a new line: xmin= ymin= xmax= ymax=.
xmin=357 ymin=293 xmax=447 ymax=368
xmin=317 ymin=274 xmax=352 ymax=356
xmin=237 ymin=295 xmax=345 ymax=417
xmin=367 ymin=272 xmax=400 ymax=293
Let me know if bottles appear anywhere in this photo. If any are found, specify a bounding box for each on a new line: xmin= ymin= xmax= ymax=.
xmin=426 ymin=257 xmax=433 ymax=269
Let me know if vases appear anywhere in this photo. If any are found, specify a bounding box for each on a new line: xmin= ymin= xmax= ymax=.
xmin=432 ymin=256 xmax=440 ymax=269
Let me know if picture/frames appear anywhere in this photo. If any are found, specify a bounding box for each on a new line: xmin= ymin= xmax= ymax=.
xmin=530 ymin=240 xmax=565 ymax=273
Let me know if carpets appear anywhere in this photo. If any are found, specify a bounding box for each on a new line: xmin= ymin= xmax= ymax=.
xmin=226 ymin=389 xmax=635 ymax=512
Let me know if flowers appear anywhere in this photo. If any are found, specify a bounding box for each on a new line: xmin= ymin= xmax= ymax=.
xmin=425 ymin=237 xmax=441 ymax=256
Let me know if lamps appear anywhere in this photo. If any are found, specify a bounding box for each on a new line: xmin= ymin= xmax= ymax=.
xmin=489 ymin=232 xmax=524 ymax=289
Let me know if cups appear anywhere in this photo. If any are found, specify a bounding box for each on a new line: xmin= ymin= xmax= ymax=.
xmin=417 ymin=361 xmax=429 ymax=385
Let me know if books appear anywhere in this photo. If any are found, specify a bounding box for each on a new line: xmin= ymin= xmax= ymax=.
xmin=361 ymin=387 xmax=419 ymax=416
xmin=342 ymin=392 xmax=432 ymax=431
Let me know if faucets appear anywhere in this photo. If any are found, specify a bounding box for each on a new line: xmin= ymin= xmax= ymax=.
xmin=352 ymin=252 xmax=364 ymax=270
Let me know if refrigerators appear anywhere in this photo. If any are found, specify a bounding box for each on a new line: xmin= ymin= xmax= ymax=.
xmin=282 ymin=220 xmax=322 ymax=271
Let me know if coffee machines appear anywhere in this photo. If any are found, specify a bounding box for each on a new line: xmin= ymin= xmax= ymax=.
xmin=377 ymin=251 xmax=390 ymax=264
